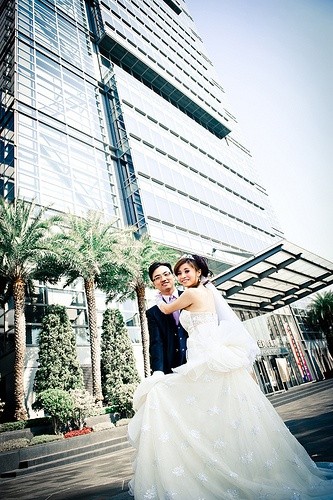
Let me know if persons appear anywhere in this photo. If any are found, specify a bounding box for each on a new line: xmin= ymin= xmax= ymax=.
xmin=143 ymin=261 xmax=190 ymax=376
xmin=125 ymin=254 xmax=333 ymax=500
